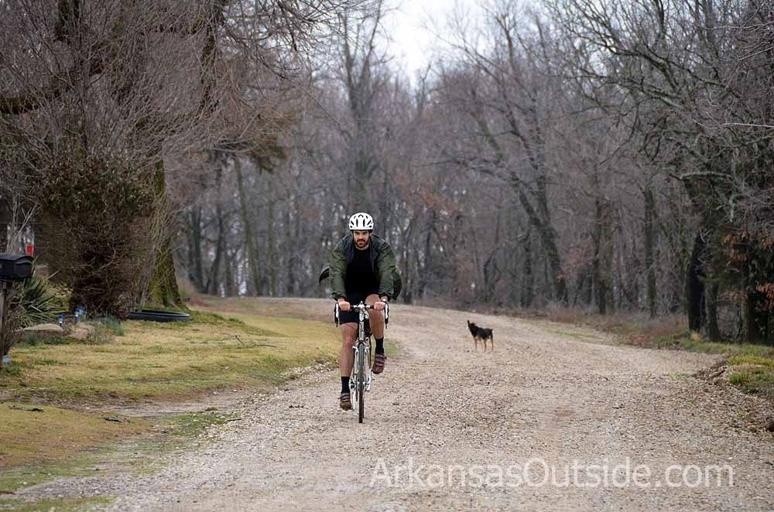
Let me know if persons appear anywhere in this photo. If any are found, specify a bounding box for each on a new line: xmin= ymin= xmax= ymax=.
xmin=329 ymin=213 xmax=396 ymax=411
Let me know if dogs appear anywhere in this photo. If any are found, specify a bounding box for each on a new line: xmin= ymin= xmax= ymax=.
xmin=467 ymin=319 xmax=494 ymax=352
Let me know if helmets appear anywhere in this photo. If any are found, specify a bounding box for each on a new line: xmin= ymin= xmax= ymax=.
xmin=349 ymin=212 xmax=374 ymax=231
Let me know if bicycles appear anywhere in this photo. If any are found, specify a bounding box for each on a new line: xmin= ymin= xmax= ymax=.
xmin=334 ymin=299 xmax=389 ymax=425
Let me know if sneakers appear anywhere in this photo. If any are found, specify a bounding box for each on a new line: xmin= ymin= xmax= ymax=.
xmin=373 ymin=354 xmax=385 ymax=373
xmin=340 ymin=393 xmax=351 ymax=410
